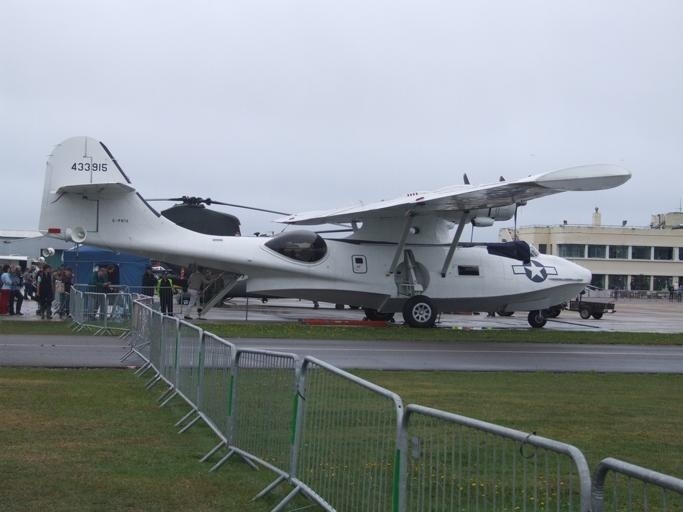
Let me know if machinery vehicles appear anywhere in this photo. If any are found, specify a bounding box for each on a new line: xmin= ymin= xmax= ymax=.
xmin=547 ymin=285 xmax=616 ymax=320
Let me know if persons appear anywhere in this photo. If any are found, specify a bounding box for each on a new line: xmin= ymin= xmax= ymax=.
xmin=157 ymin=271 xmax=175 ymax=316
xmin=142 ymin=267 xmax=157 ymax=298
xmin=183 ymin=265 xmax=213 ymax=319
xmin=105 ymin=265 xmax=116 ymax=291
xmin=0 ymin=260 xmax=73 ymax=319
xmin=677 ymin=283 xmax=682 ymax=299
xmin=669 ymin=282 xmax=675 ymax=301
xmin=89 ymin=264 xmax=107 ymax=318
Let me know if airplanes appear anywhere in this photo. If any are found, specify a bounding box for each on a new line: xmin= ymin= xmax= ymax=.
xmin=38 ymin=133 xmax=631 ymax=329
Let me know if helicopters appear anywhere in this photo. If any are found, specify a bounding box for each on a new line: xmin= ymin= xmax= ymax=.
xmin=141 ymin=195 xmax=290 ymax=308
